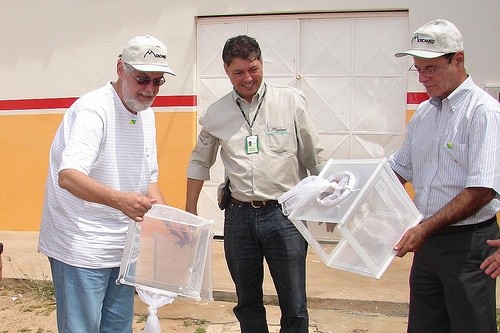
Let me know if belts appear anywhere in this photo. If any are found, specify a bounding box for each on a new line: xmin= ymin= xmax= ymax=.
xmin=437 ymin=213 xmax=497 ymax=231
xmin=228 ymin=195 xmax=282 ymax=210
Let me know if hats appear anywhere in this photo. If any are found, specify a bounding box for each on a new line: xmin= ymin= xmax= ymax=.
xmin=118 ymin=34 xmax=177 ymax=77
xmin=394 ymin=19 xmax=465 ymax=60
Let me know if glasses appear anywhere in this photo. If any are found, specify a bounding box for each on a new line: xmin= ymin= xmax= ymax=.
xmin=406 ymin=50 xmax=456 ymax=79
xmin=122 ymin=61 xmax=167 ymax=87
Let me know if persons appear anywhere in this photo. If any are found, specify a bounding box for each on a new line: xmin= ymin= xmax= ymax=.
xmin=389 ymin=19 xmax=500 ymax=333
xmin=185 ymin=36 xmax=337 ymax=333
xmin=480 ymin=239 xmax=500 ymax=278
xmin=38 ymin=35 xmax=192 ymax=333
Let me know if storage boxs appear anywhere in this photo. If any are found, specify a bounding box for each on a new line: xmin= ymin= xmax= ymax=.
xmin=116 ymin=204 xmax=215 ymax=303
xmin=286 ymin=159 xmax=424 ymax=280
